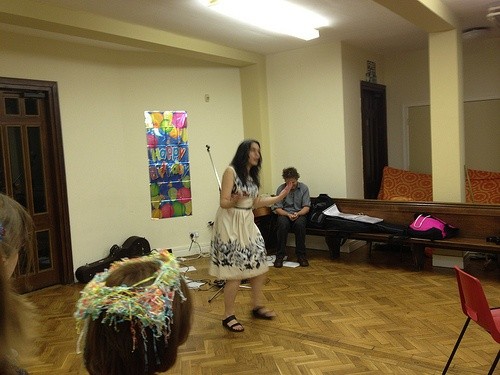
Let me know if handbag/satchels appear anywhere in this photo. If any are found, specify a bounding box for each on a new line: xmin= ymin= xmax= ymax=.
xmin=406 ymin=213 xmax=460 ymax=240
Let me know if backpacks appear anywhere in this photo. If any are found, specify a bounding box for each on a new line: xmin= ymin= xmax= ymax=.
xmin=308 ymin=193 xmax=336 ymax=228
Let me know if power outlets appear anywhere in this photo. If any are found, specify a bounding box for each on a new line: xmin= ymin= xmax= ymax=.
xmin=189 ymin=232 xmax=199 ymax=237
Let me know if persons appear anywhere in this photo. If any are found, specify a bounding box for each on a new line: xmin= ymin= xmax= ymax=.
xmin=274 ymin=167 xmax=311 ymax=268
xmin=74 ymin=248 xmax=193 ymax=375
xmin=209 ymin=138 xmax=292 ymax=332
xmin=0 ymin=193 xmax=45 ymax=375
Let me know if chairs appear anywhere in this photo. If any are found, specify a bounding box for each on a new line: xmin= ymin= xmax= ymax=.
xmin=441 ymin=265 xmax=500 ymax=375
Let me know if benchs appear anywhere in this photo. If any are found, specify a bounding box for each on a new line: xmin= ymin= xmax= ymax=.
xmin=306 ymin=197 xmax=500 ymax=272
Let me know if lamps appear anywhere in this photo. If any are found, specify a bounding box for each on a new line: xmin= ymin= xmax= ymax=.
xmin=207 ymin=0 xmax=320 ymax=41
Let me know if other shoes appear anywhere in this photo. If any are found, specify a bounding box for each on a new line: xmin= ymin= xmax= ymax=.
xmin=297 ymin=255 xmax=309 ymax=267
xmin=274 ymin=257 xmax=283 ymax=268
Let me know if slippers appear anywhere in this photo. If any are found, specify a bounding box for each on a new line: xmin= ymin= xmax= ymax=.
xmin=252 ymin=306 xmax=273 ymax=320
xmin=222 ymin=314 xmax=244 ymax=332
